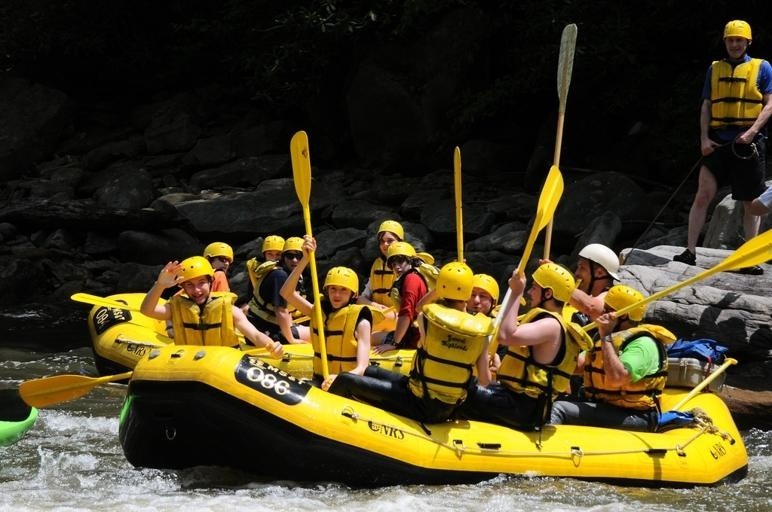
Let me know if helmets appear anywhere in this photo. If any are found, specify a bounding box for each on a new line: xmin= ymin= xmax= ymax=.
xmin=577 ymin=242 xmax=622 ymax=282
xmin=174 ymin=234 xmax=307 ymax=287
xmin=435 ymin=261 xmax=501 ymax=306
xmin=530 ymin=262 xmax=576 ymax=303
xmin=386 ymin=241 xmax=417 ymax=263
xmin=377 ymin=219 xmax=404 ymax=242
xmin=603 ymin=285 xmax=647 ymax=323
xmin=722 ymin=18 xmax=753 ymax=42
xmin=322 ymin=265 xmax=360 ymax=300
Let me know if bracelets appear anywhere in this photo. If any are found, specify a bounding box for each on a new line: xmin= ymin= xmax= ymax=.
xmin=389 ymin=340 xmax=399 ymax=346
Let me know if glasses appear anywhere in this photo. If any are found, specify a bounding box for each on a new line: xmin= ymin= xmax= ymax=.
xmin=213 ymin=256 xmax=227 ymax=263
xmin=284 ymin=252 xmax=303 ymax=261
xmin=388 ymin=254 xmax=405 ymax=268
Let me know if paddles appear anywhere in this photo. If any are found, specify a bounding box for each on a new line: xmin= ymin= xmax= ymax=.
xmin=70 ymin=292 xmax=142 ymax=313
xmin=290 ymin=131 xmax=329 ymax=379
xmin=544 ymin=24 xmax=578 ymax=259
xmin=582 ymin=229 xmax=772 ymax=332
xmin=454 ymin=146 xmax=464 ymax=262
xmin=19 ymin=371 xmax=133 ymax=407
xmin=489 ymin=165 xmax=565 ymax=357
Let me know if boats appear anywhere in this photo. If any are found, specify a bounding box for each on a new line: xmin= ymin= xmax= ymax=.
xmin=0 ymin=387 xmax=40 ymax=447
xmin=118 ymin=345 xmax=749 ymax=489
xmin=88 ymin=292 xmax=500 ymax=387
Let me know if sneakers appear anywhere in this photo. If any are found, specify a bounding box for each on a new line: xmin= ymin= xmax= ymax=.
xmin=674 ymin=253 xmax=696 ymax=265
xmin=741 ymin=264 xmax=763 ymax=275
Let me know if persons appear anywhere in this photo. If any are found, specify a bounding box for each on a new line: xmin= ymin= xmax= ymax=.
xmin=203 ymin=242 xmax=234 ymax=292
xmin=328 ymin=261 xmax=493 ymax=424
xmin=452 ymin=262 xmax=594 ymax=432
xmin=371 ymin=242 xmax=438 ymax=354
xmin=358 ymin=220 xmax=434 ymax=321
xmin=280 ymin=235 xmax=373 ymax=391
xmin=544 ymin=285 xmax=677 ymax=433
xmin=245 ymin=235 xmax=311 ymax=344
xmin=140 ymin=256 xmax=283 ymax=359
xmin=749 ymin=188 xmax=772 ymax=216
xmin=539 ymin=244 xmax=620 ymax=400
xmin=467 ymin=273 xmax=507 ymax=387
xmin=673 ymin=20 xmax=772 ymax=275
